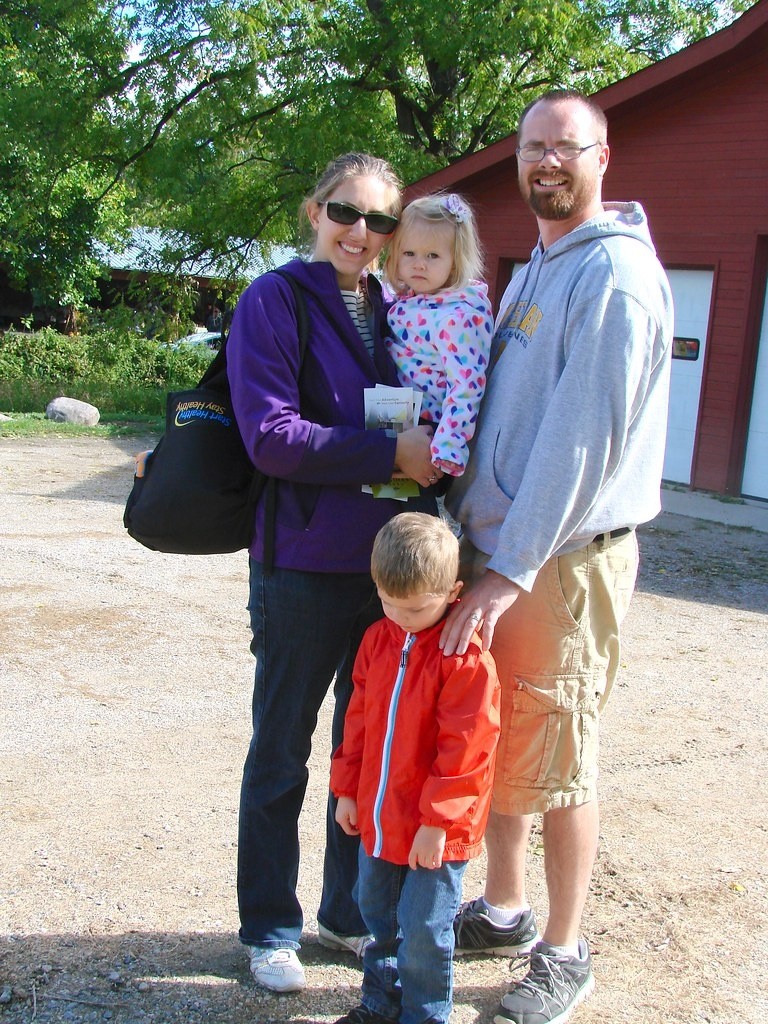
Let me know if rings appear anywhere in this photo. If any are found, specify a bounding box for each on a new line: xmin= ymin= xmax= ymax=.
xmin=469 ymin=613 xmax=480 ymax=621
xmin=428 ymin=474 xmax=436 ymax=482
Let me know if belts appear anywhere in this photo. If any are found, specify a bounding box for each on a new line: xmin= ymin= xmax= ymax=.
xmin=593 ymin=527 xmax=632 ymax=542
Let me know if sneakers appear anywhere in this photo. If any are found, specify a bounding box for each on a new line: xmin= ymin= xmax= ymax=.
xmin=493 ymin=937 xmax=594 ymax=1024
xmin=316 ymin=921 xmax=376 ymax=961
xmin=453 ymin=897 xmax=542 ymax=957
xmin=332 ymin=1003 xmax=402 ymax=1024
xmin=244 ymin=944 xmax=307 ymax=992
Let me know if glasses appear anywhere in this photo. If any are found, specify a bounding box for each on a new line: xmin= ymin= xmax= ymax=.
xmin=318 ymin=200 xmax=400 ymax=235
xmin=514 ymin=143 xmax=597 ymax=162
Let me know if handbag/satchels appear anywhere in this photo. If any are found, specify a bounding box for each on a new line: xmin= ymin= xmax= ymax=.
xmin=122 ymin=269 xmax=310 ymax=555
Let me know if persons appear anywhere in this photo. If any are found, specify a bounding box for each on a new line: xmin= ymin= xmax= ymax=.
xmin=328 ymin=514 xmax=499 ymax=1024
xmin=225 ymin=155 xmax=400 ymax=992
xmin=386 ymin=195 xmax=493 ymax=539
xmin=437 ymin=90 xmax=674 ymax=1024
